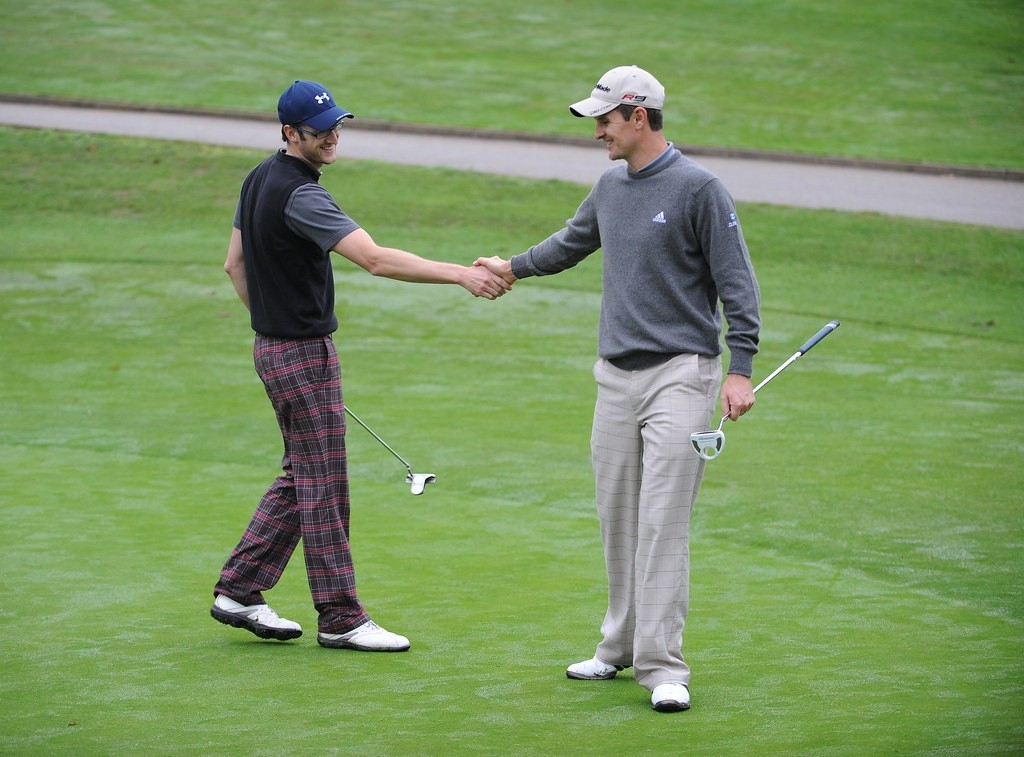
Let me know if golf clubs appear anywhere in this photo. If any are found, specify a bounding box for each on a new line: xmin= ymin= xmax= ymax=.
xmin=342 ymin=405 xmax=437 ymax=497
xmin=689 ymin=320 xmax=842 ymax=461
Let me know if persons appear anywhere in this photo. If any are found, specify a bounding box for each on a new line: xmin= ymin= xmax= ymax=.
xmin=474 ymin=65 xmax=761 ymax=713
xmin=210 ymin=79 xmax=511 ymax=653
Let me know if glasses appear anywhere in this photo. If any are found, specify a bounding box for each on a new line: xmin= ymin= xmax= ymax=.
xmin=291 ymin=120 xmax=344 ymax=139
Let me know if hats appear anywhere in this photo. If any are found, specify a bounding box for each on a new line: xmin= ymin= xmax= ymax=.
xmin=569 ymin=65 xmax=665 ymax=118
xmin=277 ymin=79 xmax=354 ymax=132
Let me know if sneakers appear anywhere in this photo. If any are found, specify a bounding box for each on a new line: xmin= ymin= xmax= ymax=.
xmin=317 ymin=619 xmax=410 ymax=652
xmin=566 ymin=650 xmax=634 ymax=680
xmin=209 ymin=593 xmax=303 ymax=641
xmin=650 ymin=682 xmax=692 ymax=711
